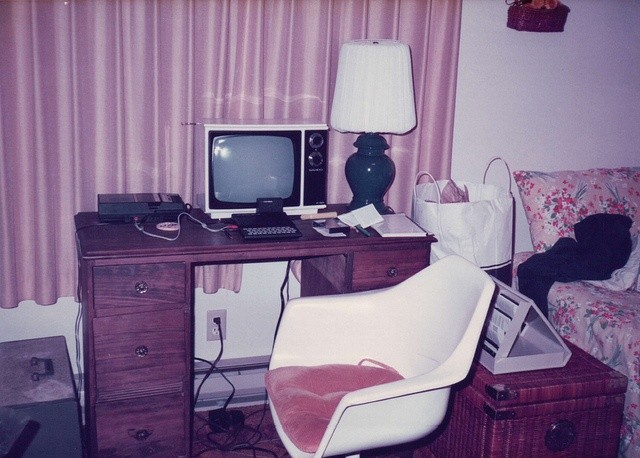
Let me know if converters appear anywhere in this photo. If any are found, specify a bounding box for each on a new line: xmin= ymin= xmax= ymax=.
xmin=208 ymin=408 xmax=245 ymax=432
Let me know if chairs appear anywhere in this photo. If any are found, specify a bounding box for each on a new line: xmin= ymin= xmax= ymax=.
xmin=266 ymin=254 xmax=493 ymax=458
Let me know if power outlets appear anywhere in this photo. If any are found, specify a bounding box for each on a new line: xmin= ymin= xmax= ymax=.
xmin=207 ymin=310 xmax=226 ymax=340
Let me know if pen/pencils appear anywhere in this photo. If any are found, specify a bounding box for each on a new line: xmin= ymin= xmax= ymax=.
xmin=355 ymin=225 xmax=371 ymax=237
xmin=352 ymin=227 xmax=359 ymax=233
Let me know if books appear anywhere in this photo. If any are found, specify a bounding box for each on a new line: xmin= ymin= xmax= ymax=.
xmin=371 ymin=213 xmax=429 ymax=237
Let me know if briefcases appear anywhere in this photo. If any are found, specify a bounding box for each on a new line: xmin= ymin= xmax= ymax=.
xmin=1 ymin=334 xmax=85 ymax=457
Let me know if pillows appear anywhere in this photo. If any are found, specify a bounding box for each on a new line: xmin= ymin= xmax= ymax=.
xmin=512 ymin=167 xmax=640 ymax=270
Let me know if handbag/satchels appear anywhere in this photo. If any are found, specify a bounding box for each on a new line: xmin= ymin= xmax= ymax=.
xmin=411 ymin=155 xmax=515 ymax=287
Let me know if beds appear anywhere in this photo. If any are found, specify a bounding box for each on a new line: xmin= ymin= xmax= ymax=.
xmin=502 ymin=248 xmax=640 ymax=457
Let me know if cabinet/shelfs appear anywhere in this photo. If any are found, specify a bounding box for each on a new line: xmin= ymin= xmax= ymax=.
xmin=407 ymin=330 xmax=629 ymax=458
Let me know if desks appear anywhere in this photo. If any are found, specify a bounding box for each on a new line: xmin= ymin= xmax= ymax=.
xmin=73 ymin=212 xmax=439 ymax=458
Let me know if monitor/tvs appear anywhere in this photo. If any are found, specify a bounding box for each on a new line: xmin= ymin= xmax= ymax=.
xmin=204 ymin=122 xmax=330 ymax=220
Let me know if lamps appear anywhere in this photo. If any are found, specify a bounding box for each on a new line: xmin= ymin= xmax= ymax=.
xmin=330 ymin=38 xmax=418 ymax=212
